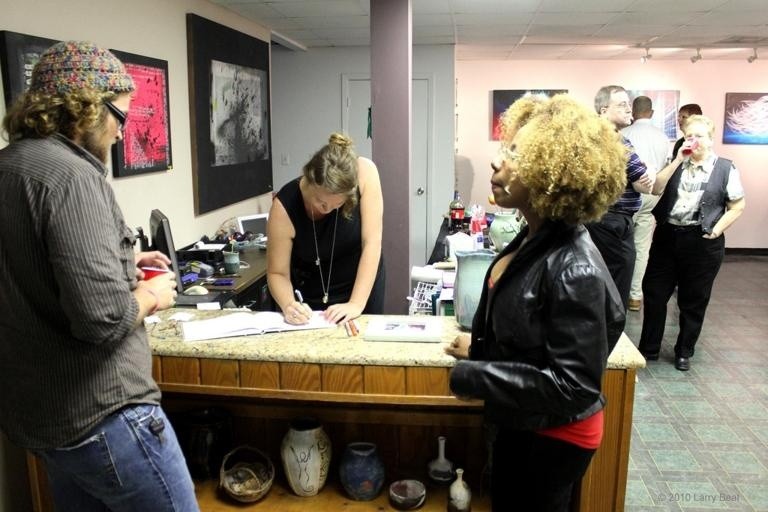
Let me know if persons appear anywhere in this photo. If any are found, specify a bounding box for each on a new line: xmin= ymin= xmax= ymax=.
xmin=619 ymin=94 xmax=673 ymax=312
xmin=584 ymin=85 xmax=653 ymax=314
xmin=0 ymin=40 xmax=200 ymax=512
xmin=444 ymin=92 xmax=632 ymax=512
xmin=670 ymin=104 xmax=703 ymax=162
xmin=639 ymin=115 xmax=745 ymax=372
xmin=266 ymin=133 xmax=384 ymax=327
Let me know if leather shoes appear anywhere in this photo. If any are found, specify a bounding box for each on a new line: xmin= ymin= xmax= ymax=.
xmin=675 ymin=354 xmax=689 ymax=371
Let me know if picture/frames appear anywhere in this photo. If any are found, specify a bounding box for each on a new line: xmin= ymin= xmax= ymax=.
xmin=108 ymin=44 xmax=175 ymax=183
xmin=0 ymin=26 xmax=72 ymax=125
xmin=181 ymin=10 xmax=276 ymax=216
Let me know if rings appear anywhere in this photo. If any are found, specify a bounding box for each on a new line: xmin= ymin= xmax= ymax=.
xmin=292 ymin=312 xmax=298 ymax=318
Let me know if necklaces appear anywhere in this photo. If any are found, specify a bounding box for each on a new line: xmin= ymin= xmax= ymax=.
xmin=311 ymin=204 xmax=339 ymax=304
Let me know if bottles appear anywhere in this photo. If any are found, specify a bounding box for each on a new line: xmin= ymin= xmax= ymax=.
xmin=471 ymin=208 xmax=486 ymax=234
xmin=449 ymin=189 xmax=465 ymax=234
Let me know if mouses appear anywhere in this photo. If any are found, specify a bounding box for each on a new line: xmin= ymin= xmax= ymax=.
xmin=183 ymin=285 xmax=209 ymax=296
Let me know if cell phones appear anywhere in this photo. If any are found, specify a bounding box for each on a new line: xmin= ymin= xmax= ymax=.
xmin=215 ymin=279 xmax=233 ymax=285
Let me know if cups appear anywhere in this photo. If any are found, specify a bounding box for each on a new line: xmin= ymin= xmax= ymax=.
xmin=141 ymin=265 xmax=167 ymax=281
xmin=683 ymin=137 xmax=700 ymax=156
xmin=222 ymin=250 xmax=240 ymax=274
xmin=482 ymin=227 xmax=490 ymax=249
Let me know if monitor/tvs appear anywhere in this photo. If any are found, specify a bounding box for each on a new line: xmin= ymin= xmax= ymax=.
xmin=235 ymin=212 xmax=269 ymax=235
xmin=135 ymin=210 xmax=184 ymax=293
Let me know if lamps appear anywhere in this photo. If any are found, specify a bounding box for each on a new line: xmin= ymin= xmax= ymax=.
xmin=687 ymin=45 xmax=702 ymax=64
xmin=638 ymin=44 xmax=653 ymax=64
xmin=745 ymin=44 xmax=759 ymax=63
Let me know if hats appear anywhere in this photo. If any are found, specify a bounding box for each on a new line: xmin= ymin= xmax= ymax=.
xmin=31 ymin=40 xmax=134 ymax=95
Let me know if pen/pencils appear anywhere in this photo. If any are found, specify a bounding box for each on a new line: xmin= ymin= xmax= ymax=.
xmin=295 ymin=288 xmax=305 ymax=306
xmin=346 ymin=319 xmax=358 ymax=336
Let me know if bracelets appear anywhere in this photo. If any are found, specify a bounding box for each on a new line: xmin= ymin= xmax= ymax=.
xmin=138 ymin=285 xmax=161 ymax=316
xmin=712 ymin=232 xmax=718 ymax=239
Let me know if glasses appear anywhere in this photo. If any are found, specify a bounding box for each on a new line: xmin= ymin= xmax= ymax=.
xmin=104 ymin=100 xmax=127 ymax=128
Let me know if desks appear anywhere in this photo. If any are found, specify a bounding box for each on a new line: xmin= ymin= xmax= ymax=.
xmin=178 ymin=225 xmax=271 ymax=303
xmin=423 ymin=209 xmax=497 ymax=275
xmin=20 ymin=302 xmax=652 ymax=512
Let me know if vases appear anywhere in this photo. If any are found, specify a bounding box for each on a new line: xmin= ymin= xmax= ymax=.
xmin=341 ymin=435 xmax=385 ymax=501
xmin=283 ymin=413 xmax=333 ymax=500
xmin=444 ymin=463 xmax=474 ymax=512
xmin=489 ymin=213 xmax=521 ymax=244
xmin=453 ymin=246 xmax=499 ymax=326
xmin=424 ymin=433 xmax=453 ymax=484
